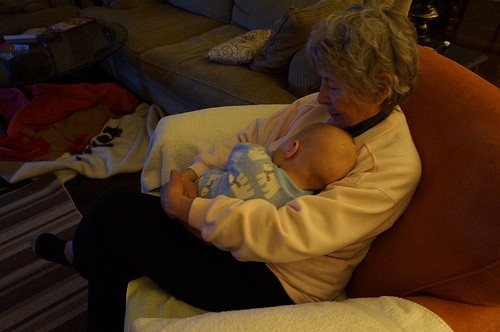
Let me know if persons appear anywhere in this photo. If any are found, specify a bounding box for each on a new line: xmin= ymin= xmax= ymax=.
xmin=193 ymin=121 xmax=358 ymax=211
xmin=33 ymin=4 xmax=422 ymax=332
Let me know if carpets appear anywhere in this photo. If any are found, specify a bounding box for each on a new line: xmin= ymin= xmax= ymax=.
xmin=0 ymin=171 xmax=91 ymax=332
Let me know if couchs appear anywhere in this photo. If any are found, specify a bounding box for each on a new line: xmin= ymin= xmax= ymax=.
xmin=124 ymin=46 xmax=500 ymax=332
xmin=0 ymin=0 xmax=411 ymax=116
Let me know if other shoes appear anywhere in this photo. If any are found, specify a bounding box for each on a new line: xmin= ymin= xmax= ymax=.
xmin=31 ymin=230 xmax=73 ymax=268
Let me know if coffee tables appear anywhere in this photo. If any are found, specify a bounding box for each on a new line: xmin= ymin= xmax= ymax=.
xmin=0 ymin=19 xmax=129 ymax=87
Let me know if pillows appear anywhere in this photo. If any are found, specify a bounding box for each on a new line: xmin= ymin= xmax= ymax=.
xmin=205 ymin=29 xmax=272 ymax=65
xmin=250 ymin=0 xmax=365 ymax=74
xmin=288 ymin=51 xmax=322 ymax=98
